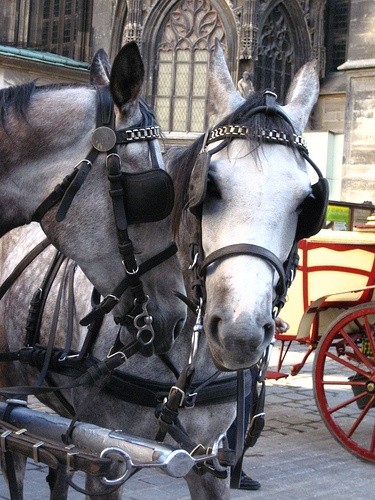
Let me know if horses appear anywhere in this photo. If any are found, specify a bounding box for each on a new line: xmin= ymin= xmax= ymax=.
xmin=1 ymin=37 xmax=323 ymax=500
xmin=0 ymin=41 xmax=189 ymax=358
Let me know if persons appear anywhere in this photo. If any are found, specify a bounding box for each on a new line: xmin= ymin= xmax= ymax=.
xmin=237 ymin=70 xmax=255 ymax=99
xmin=215 ymin=317 xmax=288 ymax=489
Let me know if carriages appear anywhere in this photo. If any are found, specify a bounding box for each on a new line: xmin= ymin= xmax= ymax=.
xmin=0 ymin=42 xmax=375 ymax=500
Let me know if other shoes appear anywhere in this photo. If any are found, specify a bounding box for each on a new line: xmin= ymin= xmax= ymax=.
xmin=240 ymin=471 xmax=261 ymax=491
